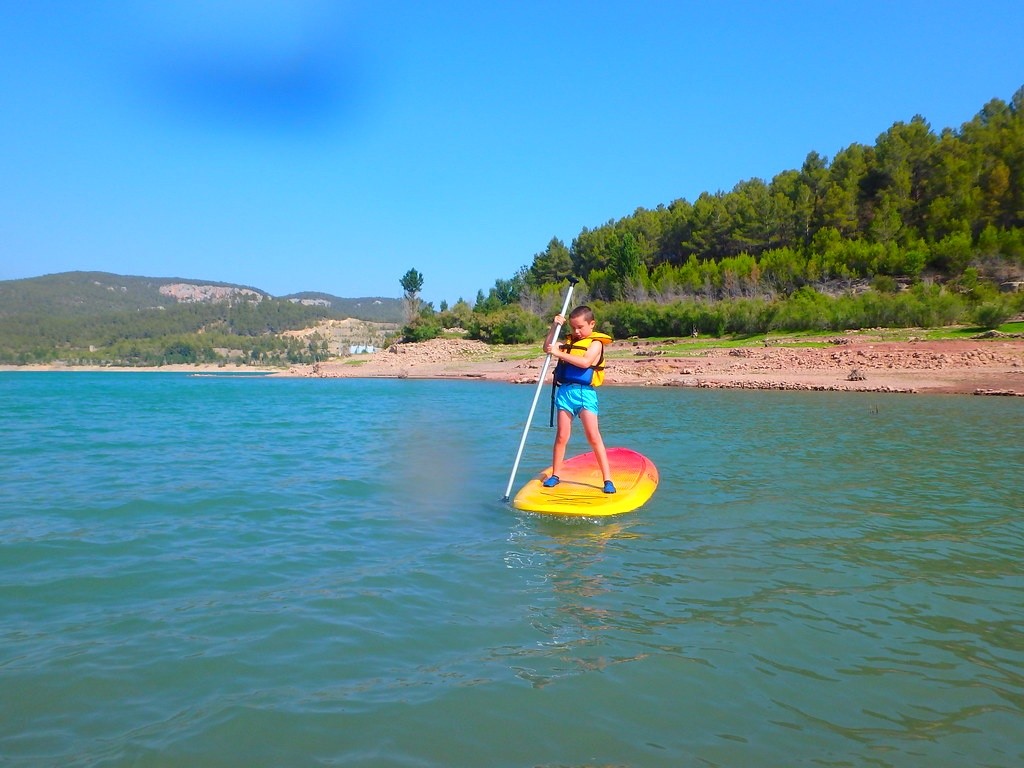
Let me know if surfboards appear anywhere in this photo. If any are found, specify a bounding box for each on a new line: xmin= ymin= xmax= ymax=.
xmin=513 ymin=447 xmax=660 ymax=515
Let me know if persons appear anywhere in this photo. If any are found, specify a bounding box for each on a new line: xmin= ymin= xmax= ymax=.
xmin=542 ymin=305 xmax=618 ymax=494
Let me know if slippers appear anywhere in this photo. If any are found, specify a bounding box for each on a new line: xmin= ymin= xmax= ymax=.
xmin=543 ymin=477 xmax=560 ymax=487
xmin=604 ymin=482 xmax=616 ymax=493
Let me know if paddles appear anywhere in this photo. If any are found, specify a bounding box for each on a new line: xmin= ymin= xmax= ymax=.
xmin=502 ymin=273 xmax=578 ymax=502
